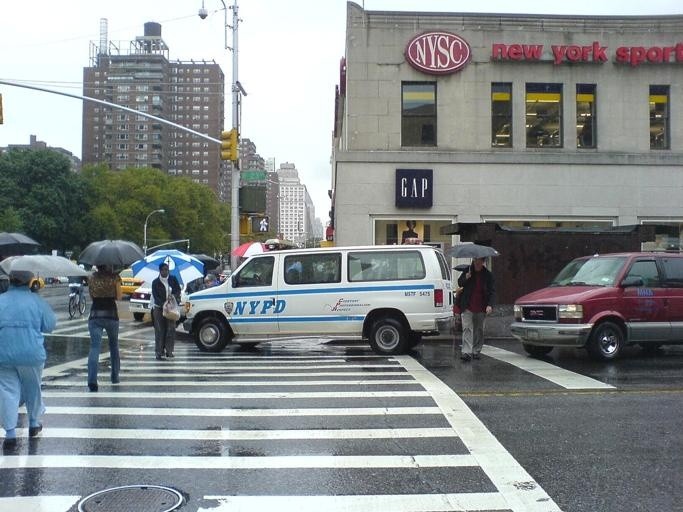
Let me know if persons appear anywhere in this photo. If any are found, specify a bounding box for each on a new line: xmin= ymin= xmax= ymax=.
xmin=152 ymin=263 xmax=181 ymax=360
xmin=87 ymin=264 xmax=122 ymax=392
xmin=67 ymin=276 xmax=89 ymax=306
xmin=458 ymin=256 xmax=494 ymax=361
xmin=0 ymin=271 xmax=56 ymax=442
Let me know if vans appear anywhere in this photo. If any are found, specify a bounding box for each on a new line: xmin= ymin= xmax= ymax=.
xmin=508 ymin=252 xmax=682 ymax=363
xmin=181 ymin=244 xmax=454 ymax=356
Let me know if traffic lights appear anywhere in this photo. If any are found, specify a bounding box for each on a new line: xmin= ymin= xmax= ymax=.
xmin=218 ymin=128 xmax=238 ymax=161
xmin=250 ymin=216 xmax=270 ymax=233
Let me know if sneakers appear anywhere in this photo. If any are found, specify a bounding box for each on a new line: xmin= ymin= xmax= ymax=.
xmin=88 ymin=382 xmax=97 ymax=392
xmin=461 ymin=354 xmax=472 ymax=361
xmin=473 ymin=354 xmax=480 ymax=358
xmin=4 ymin=439 xmax=18 ymax=450
xmin=29 ymin=423 xmax=43 ymax=437
xmin=166 ymin=353 xmax=175 ymax=357
xmin=156 ymin=354 xmax=166 ymax=361
xmin=112 ymin=378 xmax=120 ymax=383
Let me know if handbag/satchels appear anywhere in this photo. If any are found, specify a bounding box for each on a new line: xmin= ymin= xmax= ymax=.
xmin=113 ymin=273 xmax=122 ymax=300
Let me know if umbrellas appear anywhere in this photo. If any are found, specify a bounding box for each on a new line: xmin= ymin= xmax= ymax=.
xmin=227 ymin=242 xmax=270 ymax=258
xmin=0 ymin=254 xmax=89 ymax=278
xmin=445 ymin=244 xmax=501 ymax=273
xmin=452 ymin=265 xmax=472 ymax=271
xmin=188 ymin=253 xmax=220 ymax=270
xmin=131 ymin=249 xmax=204 ymax=285
xmin=0 ymin=232 xmax=39 ymax=246
xmin=77 ymin=238 xmax=144 ymax=273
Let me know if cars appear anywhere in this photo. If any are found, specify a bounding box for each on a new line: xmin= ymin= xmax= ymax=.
xmin=116 ymin=268 xmax=145 ymax=295
xmin=26 ymin=276 xmax=45 ymax=292
xmin=127 ymin=281 xmax=157 ymax=321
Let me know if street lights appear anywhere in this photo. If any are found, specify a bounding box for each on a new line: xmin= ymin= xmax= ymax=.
xmin=142 ymin=208 xmax=166 ymax=257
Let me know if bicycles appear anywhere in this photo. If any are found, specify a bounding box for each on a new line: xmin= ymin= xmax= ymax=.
xmin=66 ymin=282 xmax=87 ymax=318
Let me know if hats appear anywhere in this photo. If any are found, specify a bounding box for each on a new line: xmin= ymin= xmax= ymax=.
xmin=8 ymin=271 xmax=34 ymax=287
